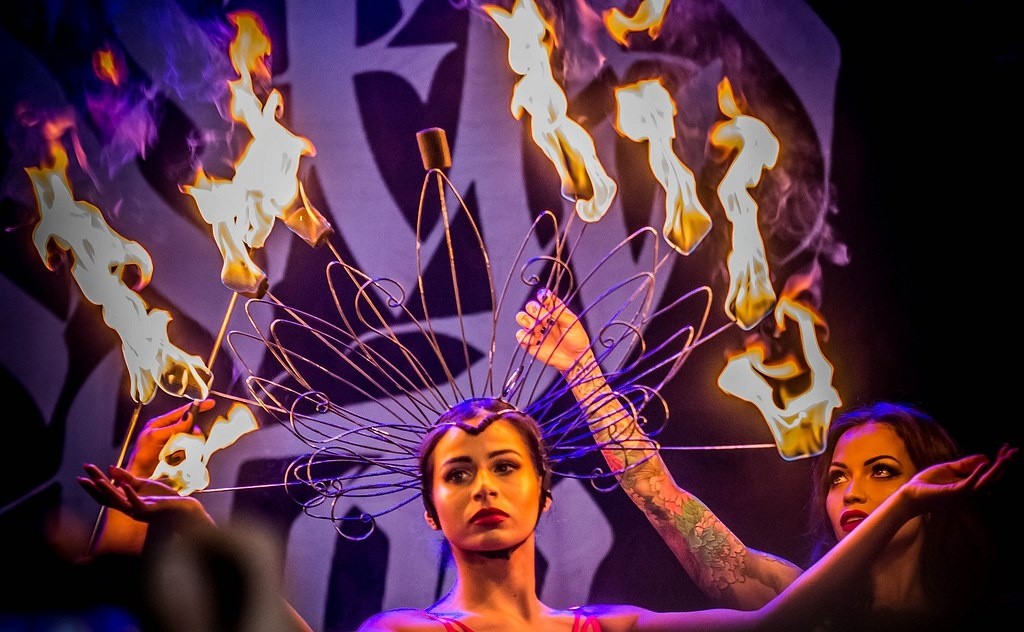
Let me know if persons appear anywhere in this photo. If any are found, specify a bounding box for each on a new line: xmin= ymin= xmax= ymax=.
xmin=74 ymin=393 xmax=1023 ymax=632
xmin=515 ymin=288 xmax=1023 ymax=632
xmin=88 ymin=397 xmax=217 ymax=557
